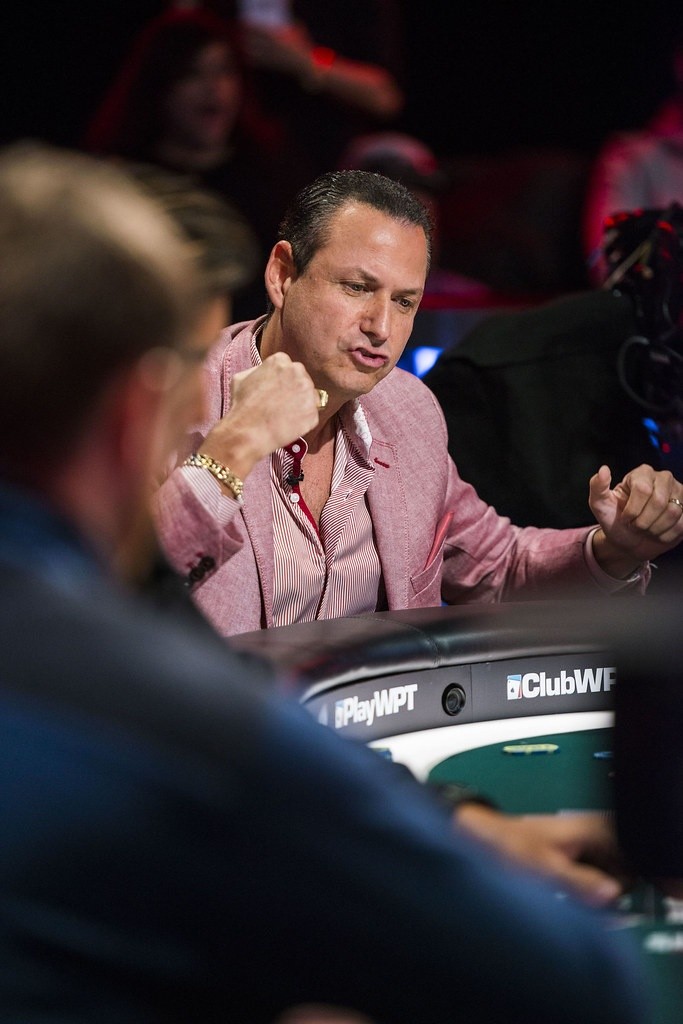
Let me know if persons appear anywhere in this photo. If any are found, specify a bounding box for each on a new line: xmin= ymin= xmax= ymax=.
xmin=0 ymin=149 xmax=683 ymax=1024
xmin=131 ymin=565 xmax=622 ymax=908
xmin=138 ymin=164 xmax=683 ymax=638
xmin=83 ymin=0 xmax=399 ymax=244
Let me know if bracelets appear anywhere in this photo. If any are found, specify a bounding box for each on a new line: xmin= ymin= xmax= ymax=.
xmin=179 ymin=452 xmax=245 ymax=500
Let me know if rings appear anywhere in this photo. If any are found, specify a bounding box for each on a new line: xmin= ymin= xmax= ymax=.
xmin=668 ymin=497 xmax=683 ymax=510
xmin=316 ymin=389 xmax=329 ymax=409
